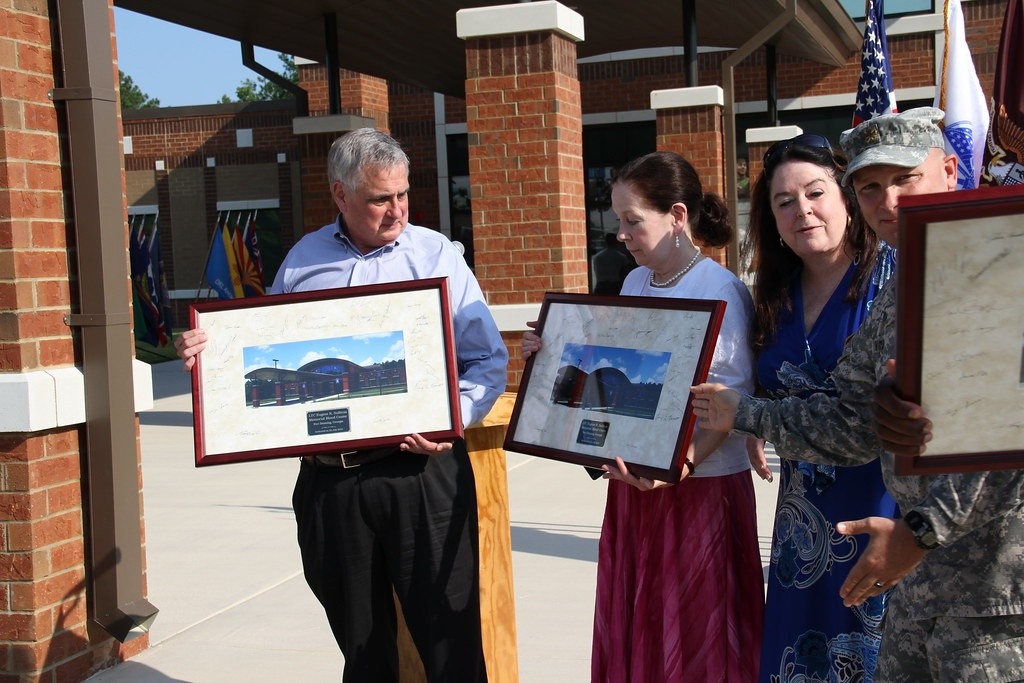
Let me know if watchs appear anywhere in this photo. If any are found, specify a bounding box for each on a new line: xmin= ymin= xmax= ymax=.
xmin=903 ymin=510 xmax=939 ymax=548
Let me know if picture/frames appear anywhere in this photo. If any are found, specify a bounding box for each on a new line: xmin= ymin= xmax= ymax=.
xmin=889 ymin=183 xmax=1024 ymax=474
xmin=503 ymin=292 xmax=731 ymax=486
xmin=189 ymin=277 xmax=464 ymax=466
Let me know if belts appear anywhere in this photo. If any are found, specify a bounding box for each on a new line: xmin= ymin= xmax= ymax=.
xmin=307 ymin=449 xmax=405 ymax=469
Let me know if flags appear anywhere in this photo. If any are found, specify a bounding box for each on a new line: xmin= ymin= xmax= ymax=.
xmin=851 ymin=0 xmax=1024 ymax=191
xmin=203 ymin=222 xmax=265 ymax=298
xmin=130 ymin=222 xmax=172 ymax=347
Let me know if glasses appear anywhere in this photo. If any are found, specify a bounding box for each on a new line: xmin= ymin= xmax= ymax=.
xmin=763 ymin=133 xmax=834 ymax=175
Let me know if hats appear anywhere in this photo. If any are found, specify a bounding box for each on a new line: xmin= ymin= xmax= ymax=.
xmin=839 ymin=106 xmax=945 ymax=160
xmin=841 ymin=117 xmax=946 ymax=187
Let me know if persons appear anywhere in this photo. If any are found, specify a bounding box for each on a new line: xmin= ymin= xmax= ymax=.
xmin=173 ymin=125 xmax=509 ymax=683
xmin=688 ymin=105 xmax=1024 ymax=682
xmin=518 ymin=150 xmax=764 ymax=683
xmin=745 ymin=133 xmax=896 ymax=682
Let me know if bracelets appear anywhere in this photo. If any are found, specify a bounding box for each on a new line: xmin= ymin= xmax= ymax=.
xmin=684 ymin=457 xmax=695 ymax=475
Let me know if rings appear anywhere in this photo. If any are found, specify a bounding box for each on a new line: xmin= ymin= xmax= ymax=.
xmin=874 ymin=582 xmax=884 ymax=588
